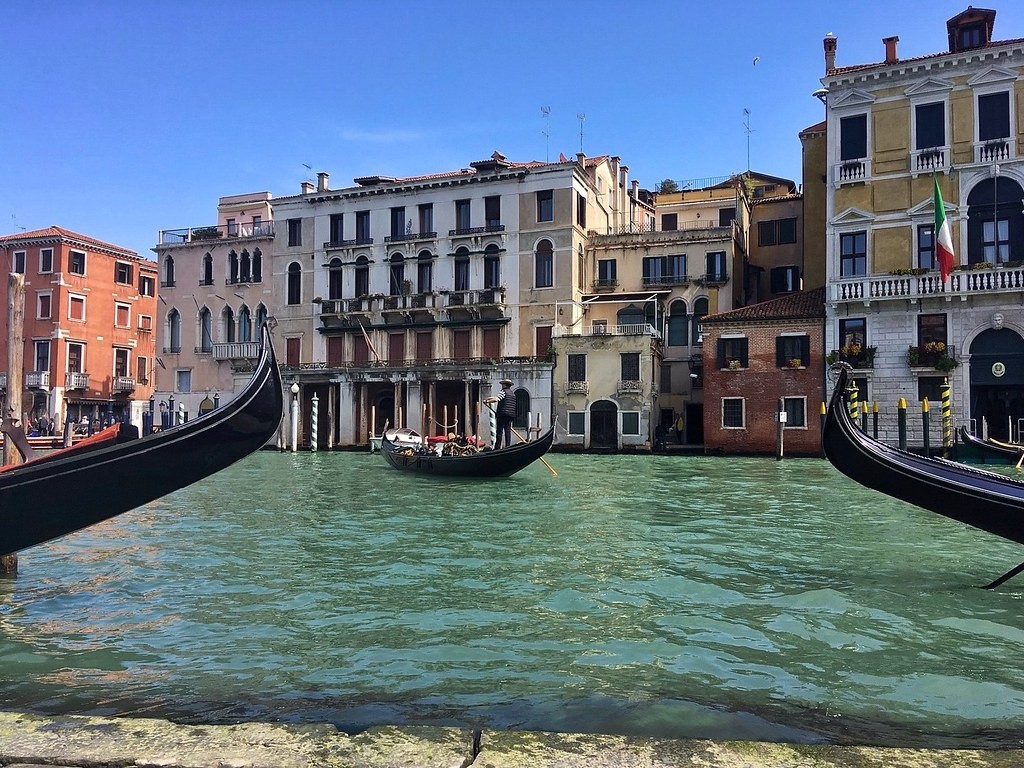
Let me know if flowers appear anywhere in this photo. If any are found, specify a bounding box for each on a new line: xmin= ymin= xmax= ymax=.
xmin=974 ymin=261 xmax=993 ymax=268
xmin=888 ymin=268 xmax=930 ymax=275
xmin=786 ymin=359 xmax=801 ymax=370
xmin=924 ymin=342 xmax=945 ymax=354
xmin=841 ymin=344 xmax=860 ymax=356
xmin=728 ymin=360 xmax=741 ymax=371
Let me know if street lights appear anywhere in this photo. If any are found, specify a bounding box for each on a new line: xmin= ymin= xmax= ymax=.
xmin=985 ymin=150 xmax=1000 ymax=290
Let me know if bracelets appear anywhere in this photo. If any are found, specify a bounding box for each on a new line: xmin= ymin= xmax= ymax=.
xmin=486 ymin=400 xmax=487 ymax=403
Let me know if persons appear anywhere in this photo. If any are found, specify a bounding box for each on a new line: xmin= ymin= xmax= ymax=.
xmin=34 ymin=416 xmax=48 ymax=436
xmin=73 ymin=415 xmax=87 ymax=434
xmin=991 ymin=313 xmax=1004 ymax=329
xmin=672 ymin=413 xmax=683 ymax=444
xmin=48 ymin=418 xmax=55 ymax=436
xmin=448 ymin=432 xmax=469 ymax=445
xmin=656 ymin=424 xmax=669 ymax=453
xmin=483 ymin=379 xmax=516 ymax=449
xmin=28 ymin=418 xmax=33 ymax=434
xmin=420 ymin=442 xmax=441 ymax=457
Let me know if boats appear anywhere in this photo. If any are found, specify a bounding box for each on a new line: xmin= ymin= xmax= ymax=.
xmin=0 ymin=320 xmax=284 ymax=559
xmin=381 ymin=413 xmax=558 ymax=478
xmin=369 ymin=430 xmax=493 ymax=458
xmin=822 ymin=367 xmax=1024 ymax=545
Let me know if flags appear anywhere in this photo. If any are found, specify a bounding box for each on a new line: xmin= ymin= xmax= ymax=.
xmin=935 ymin=178 xmax=954 ymax=282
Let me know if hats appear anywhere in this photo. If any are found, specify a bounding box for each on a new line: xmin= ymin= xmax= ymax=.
xmin=499 ymin=379 xmax=514 ymax=386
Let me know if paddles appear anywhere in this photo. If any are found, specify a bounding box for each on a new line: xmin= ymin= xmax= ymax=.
xmin=984 ymin=560 xmax=1024 ymax=587
xmin=483 ymin=399 xmax=558 ymax=476
